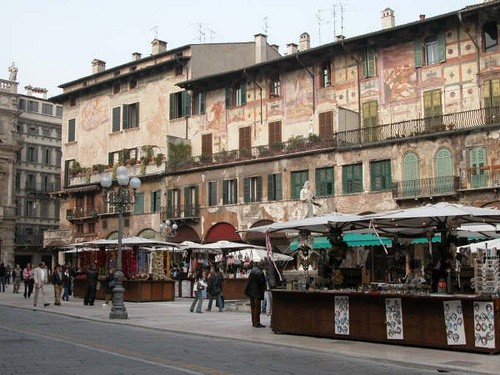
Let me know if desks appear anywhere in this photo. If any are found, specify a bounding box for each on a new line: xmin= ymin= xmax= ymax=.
xmin=72 ymin=277 xmax=175 ymax=304
xmin=269 ymin=287 xmax=500 ymax=355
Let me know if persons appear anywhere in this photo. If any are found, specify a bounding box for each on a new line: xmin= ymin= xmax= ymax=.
xmin=318 ymin=248 xmax=332 ymax=287
xmin=84 ymin=264 xmax=99 ymax=306
xmin=0 ymin=262 xmax=6 ymax=292
xmin=52 ymin=264 xmax=64 ymax=306
xmin=5 ymin=265 xmax=11 ymax=285
xmin=102 ymin=268 xmax=115 ymax=306
xmin=205 ymin=271 xmax=224 ymax=312
xmin=33 ymin=262 xmax=51 ymax=307
xmin=12 ymin=264 xmax=22 ymax=294
xmin=23 ymin=263 xmax=31 ymax=298
xmin=189 ymin=271 xmax=208 ymax=313
xmin=62 ymin=268 xmax=71 ymax=301
xmin=245 ymin=259 xmax=268 ymax=328
xmin=300 ymin=181 xmax=322 ymax=219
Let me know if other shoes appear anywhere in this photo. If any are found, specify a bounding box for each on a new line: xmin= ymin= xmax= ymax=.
xmin=84 ymin=301 xmax=88 ymax=305
xmin=219 ymin=308 xmax=223 ymax=311
xmin=102 ymin=303 xmax=110 ymax=307
xmin=66 ymin=298 xmax=69 ymax=301
xmin=190 ymin=309 xmax=194 ymax=312
xmin=252 ymin=323 xmax=256 ymax=327
xmin=61 ymin=297 xmax=65 ymax=301
xmin=44 ymin=303 xmax=50 ymax=307
xmin=196 ymin=310 xmax=202 ymax=314
xmin=256 ymin=323 xmax=266 ymax=328
xmin=206 ymin=308 xmax=211 ymax=311
xmin=58 ymin=303 xmax=61 ymax=306
xmin=89 ymin=302 xmax=94 ymax=305
xmin=55 ymin=302 xmax=58 ymax=305
xmin=34 ymin=304 xmax=36 ymax=306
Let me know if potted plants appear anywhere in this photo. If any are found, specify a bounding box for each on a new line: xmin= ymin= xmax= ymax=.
xmin=169 ymin=133 xmax=322 ymax=170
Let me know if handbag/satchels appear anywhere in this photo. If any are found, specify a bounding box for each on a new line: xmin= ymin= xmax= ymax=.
xmin=170 ymin=250 xmax=252 ymax=278
xmin=197 ymin=280 xmax=204 ymax=290
xmin=109 ymin=280 xmax=117 ymax=287
xmin=68 ymin=288 xmax=72 ymax=296
xmin=216 ymin=295 xmax=224 ymax=308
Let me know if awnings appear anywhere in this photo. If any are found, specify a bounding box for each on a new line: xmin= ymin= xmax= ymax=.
xmin=290 ymin=230 xmax=442 ymax=250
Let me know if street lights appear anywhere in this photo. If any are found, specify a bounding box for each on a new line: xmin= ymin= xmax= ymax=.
xmin=100 ymin=165 xmax=141 ymax=319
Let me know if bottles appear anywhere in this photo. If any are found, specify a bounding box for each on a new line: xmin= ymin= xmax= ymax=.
xmin=438 ymin=279 xmax=446 ymax=294
xmin=286 ymin=274 xmax=307 ymax=290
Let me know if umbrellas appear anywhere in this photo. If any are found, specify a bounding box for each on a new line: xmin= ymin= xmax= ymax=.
xmin=61 ymin=236 xmax=294 ymax=278
xmin=234 ymin=202 xmax=500 ymax=293
